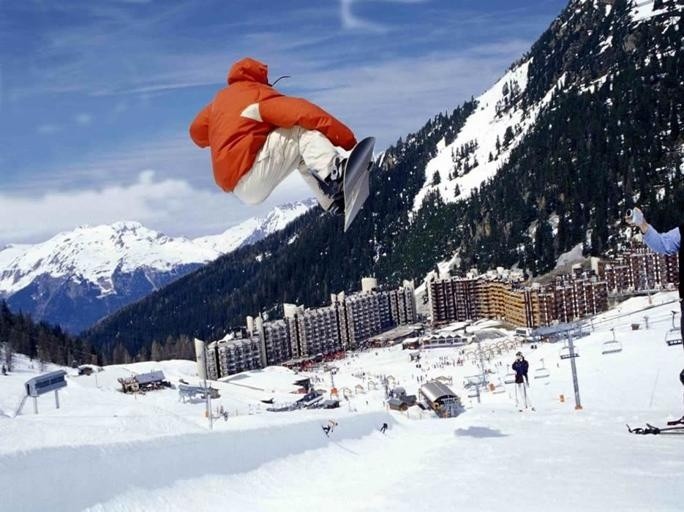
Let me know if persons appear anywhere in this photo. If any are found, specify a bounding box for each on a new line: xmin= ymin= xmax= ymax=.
xmin=189 ymin=58 xmax=359 ymax=220
xmin=624 ymin=180 xmax=684 ymax=386
xmin=380 ymin=422 xmax=388 ymax=434
xmin=321 ymin=418 xmax=338 ymax=436
xmin=511 ymin=351 xmax=536 ymax=413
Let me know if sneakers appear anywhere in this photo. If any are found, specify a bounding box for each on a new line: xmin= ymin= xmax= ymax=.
xmin=324 ymin=154 xmax=374 ymax=219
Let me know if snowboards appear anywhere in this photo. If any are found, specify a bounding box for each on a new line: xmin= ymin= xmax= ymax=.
xmin=343 ymin=136 xmax=375 ymax=232
